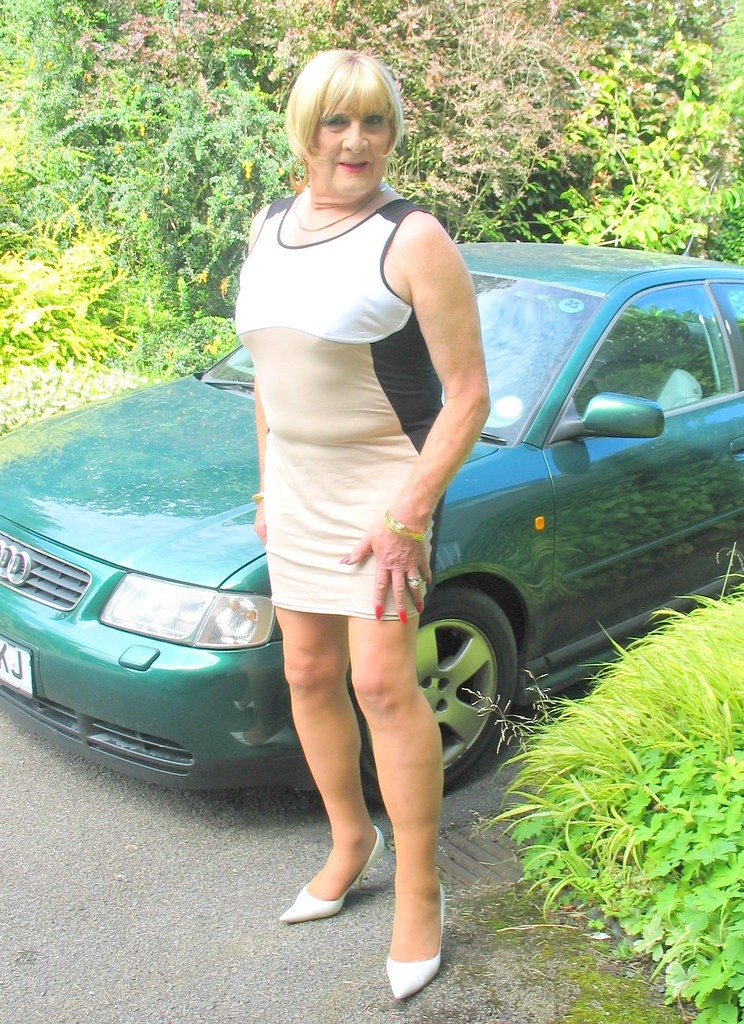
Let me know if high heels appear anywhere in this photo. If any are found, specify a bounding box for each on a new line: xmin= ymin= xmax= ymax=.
xmin=387 ymin=883 xmax=446 ymax=999
xmin=280 ymin=825 xmax=385 ymax=922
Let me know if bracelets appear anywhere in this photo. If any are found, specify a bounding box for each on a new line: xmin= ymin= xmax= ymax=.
xmin=385 ymin=510 xmax=430 ymax=541
xmin=251 ymin=492 xmax=264 ymax=503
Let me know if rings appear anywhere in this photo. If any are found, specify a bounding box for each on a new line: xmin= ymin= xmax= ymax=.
xmin=408 ymin=575 xmax=422 ymax=589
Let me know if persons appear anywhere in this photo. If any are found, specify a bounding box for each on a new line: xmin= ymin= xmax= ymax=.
xmin=234 ymin=48 xmax=490 ymax=1000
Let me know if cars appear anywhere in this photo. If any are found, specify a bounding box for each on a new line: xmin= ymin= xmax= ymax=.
xmin=1 ymin=239 xmax=744 ymax=810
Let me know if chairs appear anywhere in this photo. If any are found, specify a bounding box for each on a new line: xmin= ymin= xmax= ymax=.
xmin=599 ymin=316 xmax=696 ymax=402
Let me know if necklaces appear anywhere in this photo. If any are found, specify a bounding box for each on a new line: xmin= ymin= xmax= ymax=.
xmin=294 ymin=181 xmax=389 ymax=231
